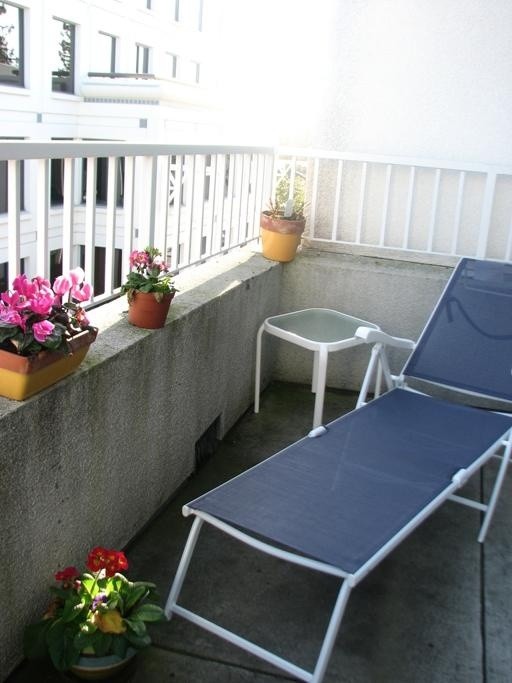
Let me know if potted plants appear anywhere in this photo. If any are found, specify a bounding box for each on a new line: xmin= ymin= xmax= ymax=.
xmin=257 ymin=176 xmax=308 ymax=264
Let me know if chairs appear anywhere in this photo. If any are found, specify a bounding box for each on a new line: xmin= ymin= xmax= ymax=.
xmin=164 ymin=255 xmax=512 ymax=682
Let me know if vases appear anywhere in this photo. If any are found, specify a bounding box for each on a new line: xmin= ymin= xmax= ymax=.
xmin=70 ymin=646 xmax=139 ymax=682
xmin=1 ymin=326 xmax=99 ymax=401
xmin=128 ymin=292 xmax=176 ymax=328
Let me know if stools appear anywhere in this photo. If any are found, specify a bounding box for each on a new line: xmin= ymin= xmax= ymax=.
xmin=253 ymin=305 xmax=381 ymax=430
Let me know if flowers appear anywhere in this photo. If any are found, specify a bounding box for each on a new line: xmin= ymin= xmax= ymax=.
xmin=23 ymin=546 xmax=164 ymax=675
xmin=119 ymin=244 xmax=181 ymax=306
xmin=1 ymin=266 xmax=93 ymax=358
